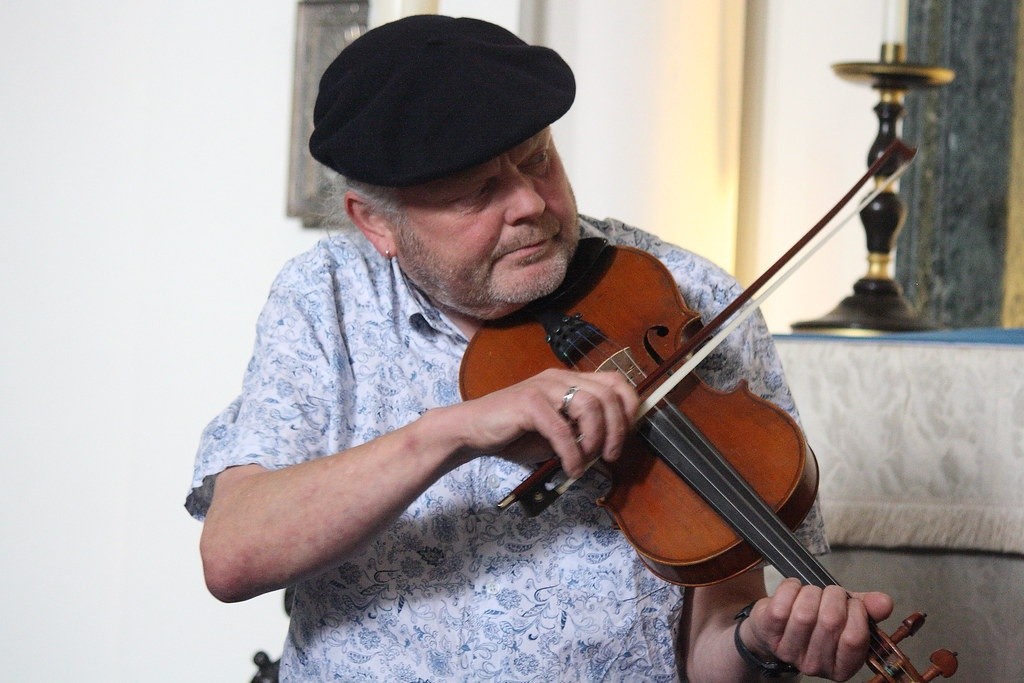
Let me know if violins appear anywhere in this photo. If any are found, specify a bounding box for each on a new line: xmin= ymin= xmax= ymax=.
xmin=458 ymin=245 xmax=960 ymax=682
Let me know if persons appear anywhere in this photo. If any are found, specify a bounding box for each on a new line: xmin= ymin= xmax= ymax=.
xmin=185 ymin=17 xmax=892 ymax=682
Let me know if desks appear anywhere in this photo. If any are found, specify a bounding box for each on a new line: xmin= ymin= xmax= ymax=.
xmin=769 ymin=327 xmax=1024 ymax=555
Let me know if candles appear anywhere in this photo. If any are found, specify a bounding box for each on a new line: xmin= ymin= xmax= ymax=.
xmin=885 ymin=0 xmax=908 ymax=44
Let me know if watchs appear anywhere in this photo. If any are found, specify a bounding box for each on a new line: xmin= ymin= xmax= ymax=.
xmin=735 ymin=598 xmax=804 ymax=683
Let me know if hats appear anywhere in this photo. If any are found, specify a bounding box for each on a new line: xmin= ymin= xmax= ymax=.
xmin=309 ymin=14 xmax=577 ymax=187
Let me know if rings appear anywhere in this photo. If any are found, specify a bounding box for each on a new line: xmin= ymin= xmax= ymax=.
xmin=562 ymin=386 xmax=579 ymax=412
